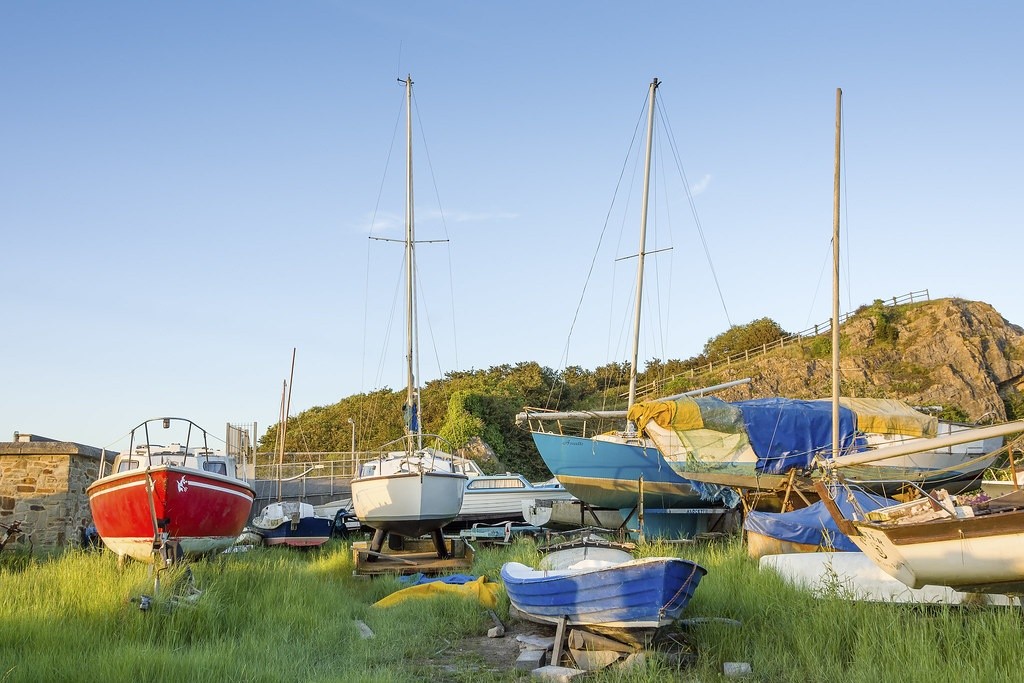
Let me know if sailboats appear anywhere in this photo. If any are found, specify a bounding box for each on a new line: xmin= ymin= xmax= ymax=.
xmin=250 ymin=348 xmax=335 ymax=549
xmin=348 ymin=73 xmax=469 ymax=541
xmin=513 ymin=77 xmax=1005 ymax=513
xmin=743 ymin=85 xmax=909 ymax=562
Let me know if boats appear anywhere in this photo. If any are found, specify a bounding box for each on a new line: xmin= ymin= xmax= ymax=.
xmin=230 ymin=527 xmax=266 ymax=546
xmin=808 ymin=413 xmax=1024 ymax=593
xmin=762 ymin=551 xmax=1019 ymax=607
xmin=498 ymin=525 xmax=708 ymax=649
xmin=88 ymin=416 xmax=255 ymax=566
xmin=311 ymin=496 xmax=367 ymax=534
xmin=450 ymin=472 xmax=743 ymax=545
